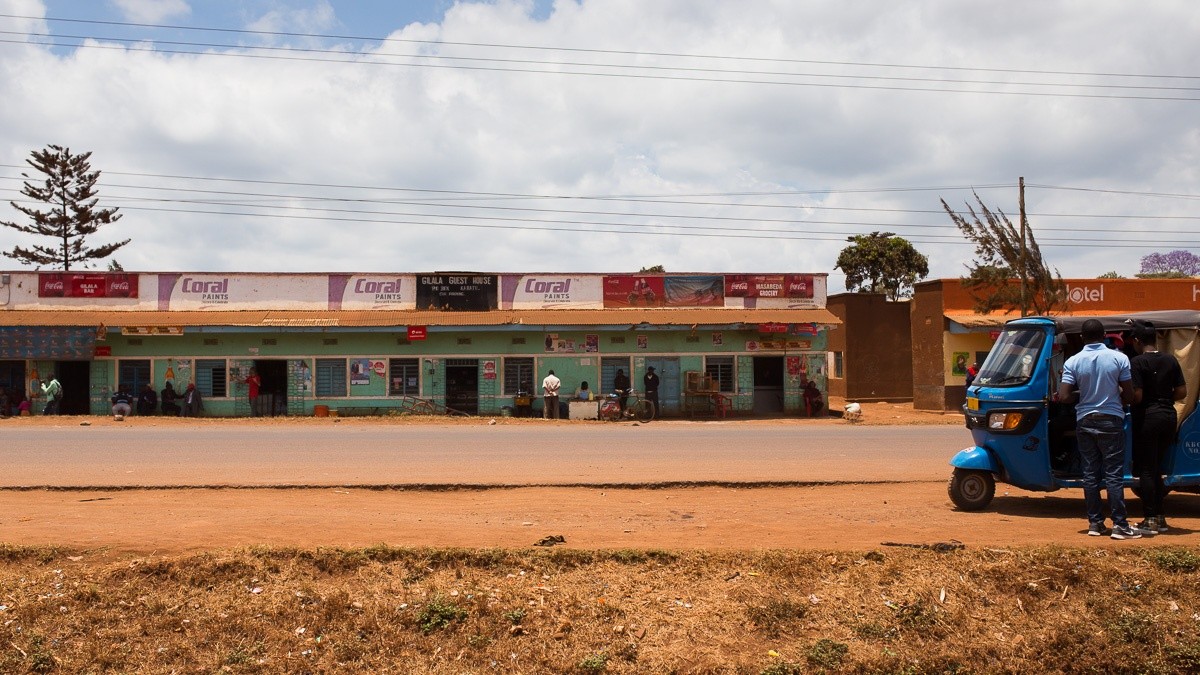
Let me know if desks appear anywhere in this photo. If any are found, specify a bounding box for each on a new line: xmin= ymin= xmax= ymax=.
xmin=568 ymin=400 xmax=599 ymax=420
xmin=684 ymin=391 xmax=717 ymax=421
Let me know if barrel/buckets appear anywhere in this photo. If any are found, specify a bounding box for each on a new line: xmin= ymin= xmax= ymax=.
xmin=559 ymin=402 xmax=568 ymax=419
xmin=328 ymin=410 xmax=339 ymax=418
xmin=500 ymin=409 xmax=512 ymax=417
xmin=314 ymin=405 xmax=328 ymax=417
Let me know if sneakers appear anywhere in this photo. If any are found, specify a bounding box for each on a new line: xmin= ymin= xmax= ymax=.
xmin=1111 ymin=522 xmax=1143 ymax=539
xmin=1130 ymin=520 xmax=1159 ymax=535
xmin=1087 ymin=523 xmax=1108 ymax=536
xmin=1157 ymin=517 xmax=1168 ymax=533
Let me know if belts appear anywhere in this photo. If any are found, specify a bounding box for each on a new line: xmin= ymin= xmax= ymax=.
xmin=1087 ymin=414 xmax=1113 ymax=419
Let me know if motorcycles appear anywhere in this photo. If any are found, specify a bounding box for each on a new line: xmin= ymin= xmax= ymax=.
xmin=946 ymin=309 xmax=1200 ymax=512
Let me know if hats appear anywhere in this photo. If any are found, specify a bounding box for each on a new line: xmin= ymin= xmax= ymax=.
xmin=648 ymin=366 xmax=656 ymax=370
xmin=187 ymin=384 xmax=194 ymax=389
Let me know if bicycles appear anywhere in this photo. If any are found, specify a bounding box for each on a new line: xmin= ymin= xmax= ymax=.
xmin=601 ymin=388 xmax=656 ymax=423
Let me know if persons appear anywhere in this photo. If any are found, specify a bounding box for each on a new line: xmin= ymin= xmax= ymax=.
xmin=161 ymin=381 xmax=184 ymax=417
xmin=16 ymin=396 xmax=32 ymax=416
xmin=40 ymin=372 xmax=62 ymax=415
xmin=546 ymin=335 xmax=552 ymax=351
xmin=803 ymin=381 xmax=824 ymax=416
xmin=239 ymin=367 xmax=261 ymax=417
xmin=1123 ymin=320 xmax=1187 ymax=535
xmin=966 ymin=362 xmax=979 ymax=389
xmin=644 ymin=366 xmax=660 ymax=418
xmin=179 ymin=384 xmax=202 ymax=417
xmin=136 ymin=384 xmax=158 ymax=416
xmin=111 ymin=384 xmax=133 ymax=416
xmin=542 ymin=370 xmax=561 ymax=419
xmin=575 ymin=381 xmax=595 ymax=400
xmin=614 ymin=369 xmax=630 ymax=410
xmin=1056 ymin=319 xmax=1145 ymax=539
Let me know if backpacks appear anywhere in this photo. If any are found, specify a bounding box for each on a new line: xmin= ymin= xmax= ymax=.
xmin=52 ymin=381 xmax=63 ymax=401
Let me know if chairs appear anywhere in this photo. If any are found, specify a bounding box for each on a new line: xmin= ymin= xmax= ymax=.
xmin=711 ymin=394 xmax=733 ymax=419
xmin=802 ymin=392 xmax=824 ymax=417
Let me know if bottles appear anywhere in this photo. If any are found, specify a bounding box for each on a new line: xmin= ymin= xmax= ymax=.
xmin=588 ymin=391 xmax=593 ymax=401
